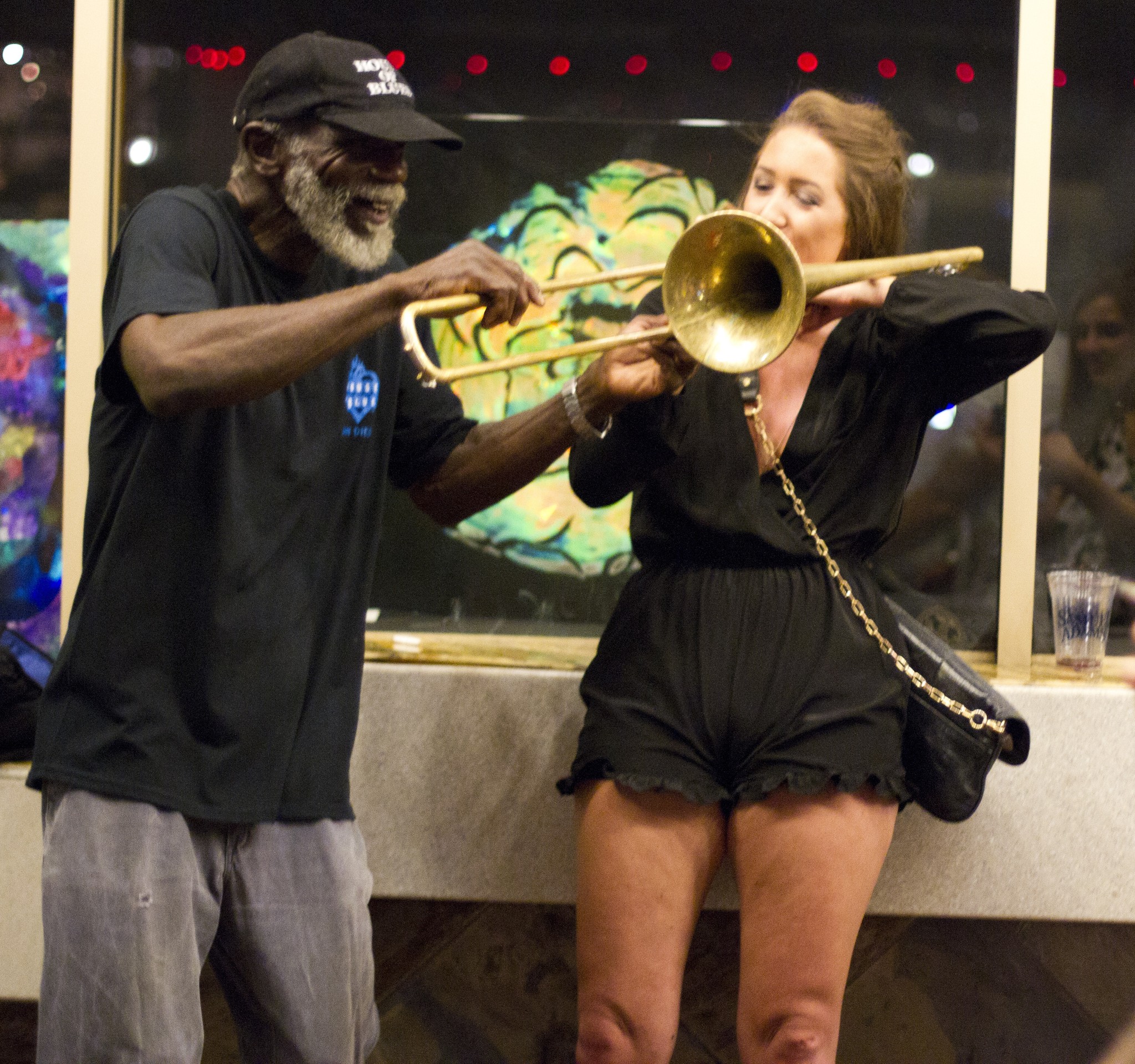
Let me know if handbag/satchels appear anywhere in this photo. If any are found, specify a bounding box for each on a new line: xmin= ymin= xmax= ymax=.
xmin=879 ymin=597 xmax=1031 ymax=824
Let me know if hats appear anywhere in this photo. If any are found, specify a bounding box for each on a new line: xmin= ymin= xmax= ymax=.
xmin=233 ymin=32 xmax=464 ymax=151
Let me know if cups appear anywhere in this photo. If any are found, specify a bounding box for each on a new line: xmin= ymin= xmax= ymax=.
xmin=1047 ymin=567 xmax=1119 ymax=668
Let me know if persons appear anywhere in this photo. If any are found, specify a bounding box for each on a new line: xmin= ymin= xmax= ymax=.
xmin=557 ymin=90 xmax=1064 ymax=1064
xmin=24 ymin=31 xmax=703 ymax=1064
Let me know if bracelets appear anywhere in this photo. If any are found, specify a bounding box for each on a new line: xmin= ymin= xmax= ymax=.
xmin=562 ymin=377 xmax=611 ymax=448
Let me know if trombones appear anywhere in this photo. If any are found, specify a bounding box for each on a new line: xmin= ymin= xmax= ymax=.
xmin=401 ymin=208 xmax=983 ymax=388
